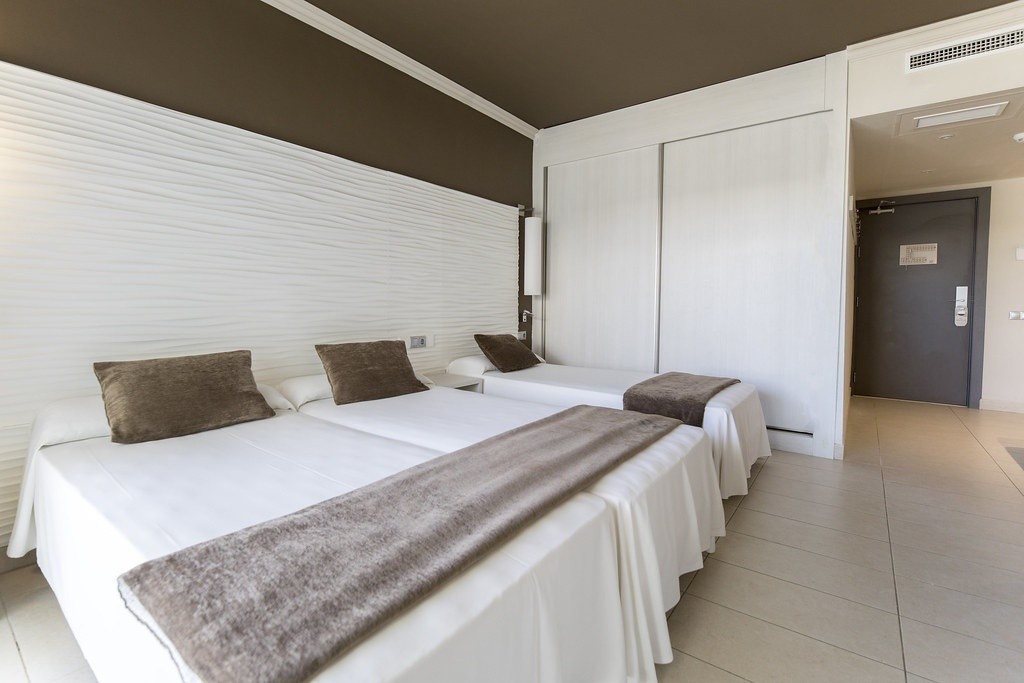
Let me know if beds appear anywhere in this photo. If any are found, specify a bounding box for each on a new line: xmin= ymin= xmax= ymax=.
xmin=5 ymin=335 xmax=773 ymax=683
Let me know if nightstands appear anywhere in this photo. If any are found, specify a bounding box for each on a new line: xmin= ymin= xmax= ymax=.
xmin=423 ymin=374 xmax=483 ymax=394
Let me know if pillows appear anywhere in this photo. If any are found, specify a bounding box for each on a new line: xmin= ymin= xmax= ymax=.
xmin=314 ymin=339 xmax=430 ymax=406
xmin=93 ymin=350 xmax=276 ymax=444
xmin=474 ymin=334 xmax=541 ymax=373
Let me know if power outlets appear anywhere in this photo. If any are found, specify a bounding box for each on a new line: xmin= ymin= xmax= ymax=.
xmin=519 ymin=330 xmax=527 ymax=341
xmin=523 ymin=312 xmax=527 ymax=323
xmin=409 ymin=336 xmax=427 ymax=349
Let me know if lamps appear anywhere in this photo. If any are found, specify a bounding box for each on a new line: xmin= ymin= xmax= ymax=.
xmin=519 ymin=206 xmax=543 ymax=295
